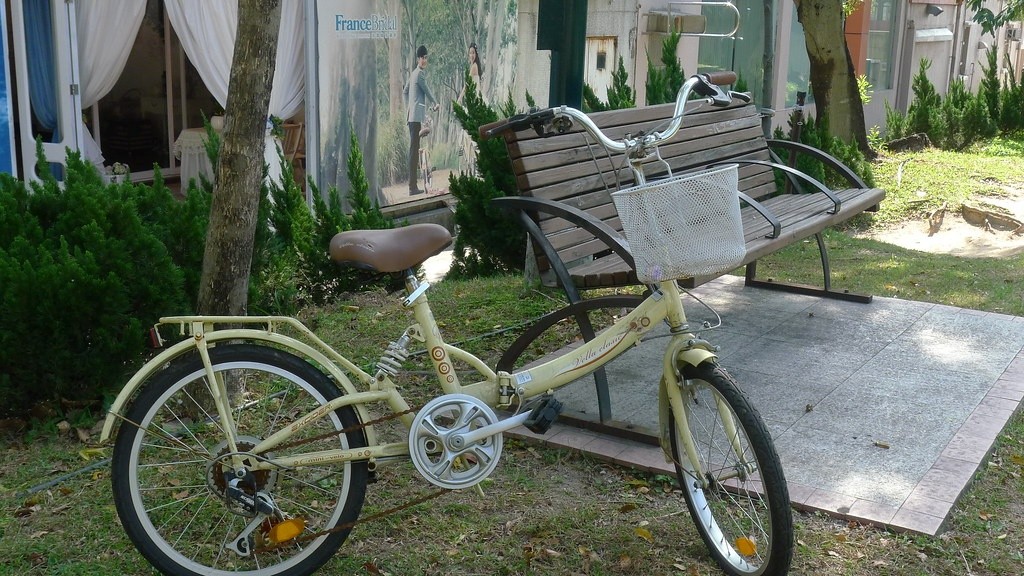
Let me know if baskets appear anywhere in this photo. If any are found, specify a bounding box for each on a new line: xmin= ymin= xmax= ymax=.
xmin=610 ymin=163 xmax=747 ymax=284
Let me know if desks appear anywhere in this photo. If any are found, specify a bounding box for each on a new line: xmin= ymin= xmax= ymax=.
xmin=174 ymin=127 xmax=215 ymax=197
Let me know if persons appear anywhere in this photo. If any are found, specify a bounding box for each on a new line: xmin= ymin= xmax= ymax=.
xmin=456 ymin=42 xmax=490 ymax=113
xmin=403 ymin=46 xmax=438 ymax=196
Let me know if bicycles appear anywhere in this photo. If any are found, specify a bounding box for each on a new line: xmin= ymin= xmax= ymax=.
xmin=412 ymin=100 xmax=441 ymax=196
xmin=449 ymin=105 xmax=481 ymax=179
xmin=87 ymin=72 xmax=797 ymax=576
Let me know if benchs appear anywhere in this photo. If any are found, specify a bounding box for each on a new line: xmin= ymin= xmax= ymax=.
xmin=497 ymin=87 xmax=887 ymax=446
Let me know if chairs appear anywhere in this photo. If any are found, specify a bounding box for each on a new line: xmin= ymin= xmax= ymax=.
xmin=277 ymin=121 xmax=306 ymax=177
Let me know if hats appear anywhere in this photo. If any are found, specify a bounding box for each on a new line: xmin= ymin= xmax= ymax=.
xmin=417 ymin=45 xmax=428 ymax=57
